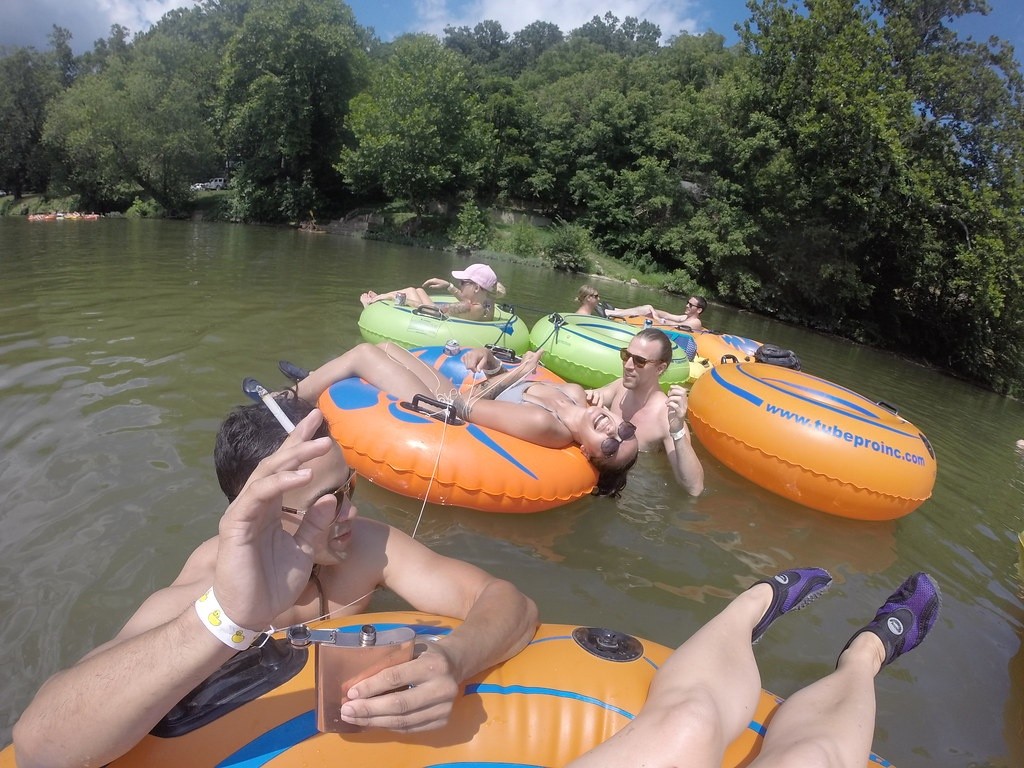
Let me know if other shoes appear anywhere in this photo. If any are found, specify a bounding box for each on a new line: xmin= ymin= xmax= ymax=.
xmin=746 ymin=567 xmax=832 ymax=642
xmin=594 ymin=301 xmax=615 ymax=318
xmin=277 ymin=359 xmax=309 ymax=384
xmin=835 ymin=570 xmax=943 ymax=678
xmin=242 ymin=376 xmax=279 ymax=404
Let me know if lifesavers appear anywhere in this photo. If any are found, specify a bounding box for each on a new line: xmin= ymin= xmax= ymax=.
xmin=1 ymin=610 xmax=900 ymax=768
xmin=315 ymin=292 xmax=938 ymax=523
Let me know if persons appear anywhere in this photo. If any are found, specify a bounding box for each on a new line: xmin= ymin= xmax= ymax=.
xmin=243 ymin=340 xmax=639 ymax=498
xmin=66 ymin=210 xmax=85 ymax=216
xmin=584 ymin=328 xmax=705 ymax=497
xmin=594 ymin=296 xmax=707 ymax=330
xmin=575 ymin=285 xmax=601 ymax=315
xmin=360 ymin=263 xmax=506 ymax=321
xmin=11 ymin=397 xmax=539 ymax=768
xmin=565 ymin=567 xmax=942 ymax=768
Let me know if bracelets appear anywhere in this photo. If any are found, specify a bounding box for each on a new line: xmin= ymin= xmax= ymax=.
xmin=482 ymin=356 xmax=503 ymax=375
xmin=447 ymin=283 xmax=454 ymax=292
xmin=452 ymin=289 xmax=459 ymax=296
xmin=194 ymin=585 xmax=262 ymax=651
xmin=670 ymin=428 xmax=686 ymax=441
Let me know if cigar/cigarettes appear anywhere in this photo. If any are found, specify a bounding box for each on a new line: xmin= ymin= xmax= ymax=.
xmin=256 ymin=385 xmax=295 ymax=437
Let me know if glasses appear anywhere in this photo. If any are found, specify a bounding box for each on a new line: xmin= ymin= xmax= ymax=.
xmin=620 ymin=347 xmax=665 ymax=368
xmin=461 ymin=281 xmax=474 ymax=285
xmin=687 ymin=301 xmax=700 ymax=309
xmin=281 ymin=467 xmax=357 ymax=528
xmin=591 ymin=294 xmax=599 ymax=299
xmin=601 ymin=421 xmax=637 ymax=460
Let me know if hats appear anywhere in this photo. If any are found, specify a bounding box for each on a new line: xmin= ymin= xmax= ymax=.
xmin=451 ymin=263 xmax=498 ymax=294
